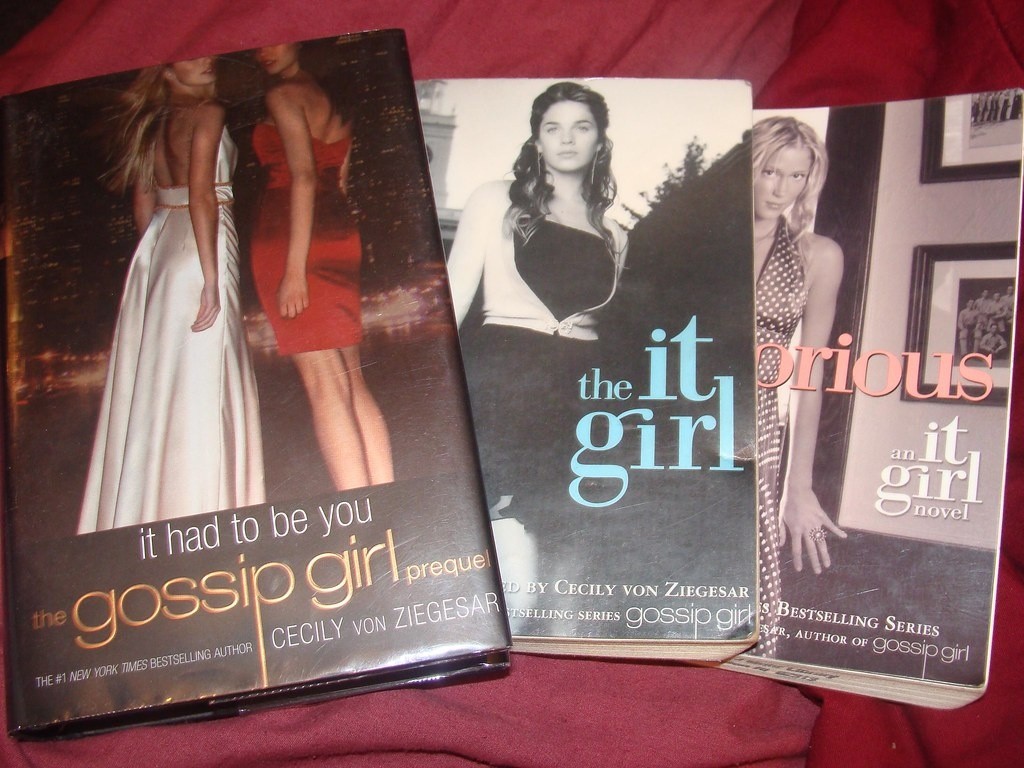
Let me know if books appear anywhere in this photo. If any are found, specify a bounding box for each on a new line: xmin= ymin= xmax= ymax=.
xmin=413 ymin=76 xmax=761 ymax=662
xmin=1 ymin=26 xmax=514 ymax=740
xmin=683 ymin=88 xmax=1024 ymax=710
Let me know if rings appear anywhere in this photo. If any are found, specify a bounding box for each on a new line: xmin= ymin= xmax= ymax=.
xmin=809 ymin=528 xmax=827 ymax=542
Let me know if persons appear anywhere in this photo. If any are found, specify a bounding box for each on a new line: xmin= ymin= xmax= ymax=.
xmin=972 ymin=89 xmax=1018 ymax=122
xmin=748 ymin=116 xmax=846 ymax=657
xmin=244 ymin=35 xmax=394 ymax=490
xmin=959 ymin=285 xmax=1014 ymax=358
xmin=77 ymin=55 xmax=264 ymax=534
xmin=446 ymin=82 xmax=628 ymax=637
